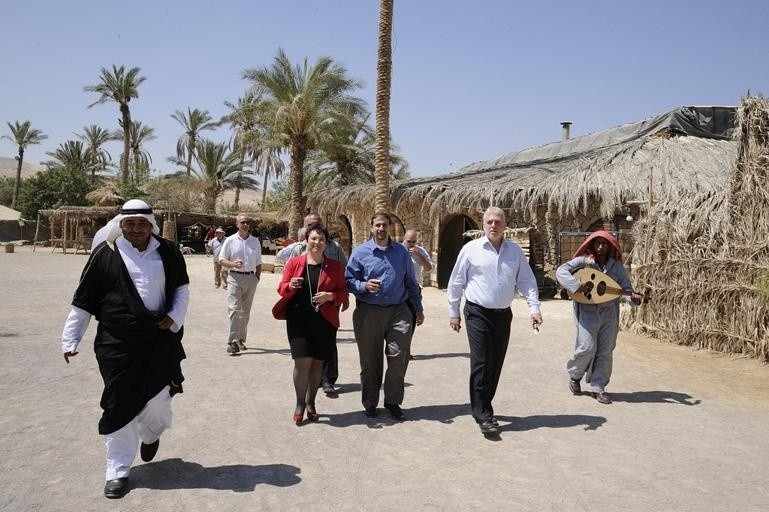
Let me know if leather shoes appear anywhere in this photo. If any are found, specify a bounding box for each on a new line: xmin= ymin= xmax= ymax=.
xmin=293 ymin=403 xmax=306 ymax=422
xmin=569 ymin=377 xmax=581 ymax=395
xmin=592 ymin=392 xmax=611 ymax=404
xmin=307 ymin=404 xmax=318 ymax=420
xmin=141 ymin=439 xmax=159 ymax=462
xmin=479 ymin=417 xmax=503 ymax=434
xmin=105 ymin=478 xmax=129 ymax=499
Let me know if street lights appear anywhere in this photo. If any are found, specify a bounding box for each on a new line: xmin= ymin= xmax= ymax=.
xmin=614 ymin=204 xmax=633 ymax=246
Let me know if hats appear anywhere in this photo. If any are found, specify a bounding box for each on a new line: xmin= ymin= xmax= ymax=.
xmin=215 ymin=228 xmax=225 ymax=234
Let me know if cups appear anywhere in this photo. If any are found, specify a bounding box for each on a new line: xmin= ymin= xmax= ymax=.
xmin=370 ymin=280 xmax=381 ymax=293
xmin=293 ymin=278 xmax=304 ymax=288
xmin=238 ymin=260 xmax=243 ymax=266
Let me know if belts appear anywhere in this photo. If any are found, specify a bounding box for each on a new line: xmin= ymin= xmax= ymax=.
xmin=230 ymin=270 xmax=254 ymax=275
xmin=466 ymin=299 xmax=511 ymax=313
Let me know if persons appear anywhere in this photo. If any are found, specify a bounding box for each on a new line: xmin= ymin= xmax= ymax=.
xmin=60 ymin=197 xmax=190 ymax=499
xmin=447 ymin=206 xmax=544 ymax=435
xmin=400 ymin=229 xmax=436 ymax=360
xmin=270 ymin=212 xmax=349 ymax=425
xmin=344 ymin=212 xmax=425 ymax=419
xmin=203 ymin=227 xmax=216 ymax=243
xmin=555 ymin=230 xmax=643 ymax=404
xmin=218 ymin=211 xmax=263 ymax=354
xmin=205 ymin=227 xmax=229 ymax=290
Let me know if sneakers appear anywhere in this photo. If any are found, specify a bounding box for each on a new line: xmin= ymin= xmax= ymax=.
xmin=328 ymin=393 xmax=338 ymax=398
xmin=215 ymin=284 xmax=220 ymax=288
xmin=237 ymin=341 xmax=247 ymax=351
xmin=221 ymin=284 xmax=227 ymax=288
xmin=365 ymin=407 xmax=377 ymax=418
xmin=227 ymin=342 xmax=239 ymax=352
xmin=386 ymin=405 xmax=404 ymax=419
xmin=323 ymin=383 xmax=335 ymax=393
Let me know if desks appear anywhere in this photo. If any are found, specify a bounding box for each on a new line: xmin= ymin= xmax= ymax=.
xmin=49 ymin=239 xmax=89 ymax=255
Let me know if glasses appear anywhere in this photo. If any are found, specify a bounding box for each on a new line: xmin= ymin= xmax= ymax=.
xmin=406 ymin=241 xmax=417 ymax=243
xmin=240 ymin=221 xmax=250 ymax=224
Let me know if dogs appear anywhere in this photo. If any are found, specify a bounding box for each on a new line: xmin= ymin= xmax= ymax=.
xmin=179 ymin=243 xmax=197 ymax=257
